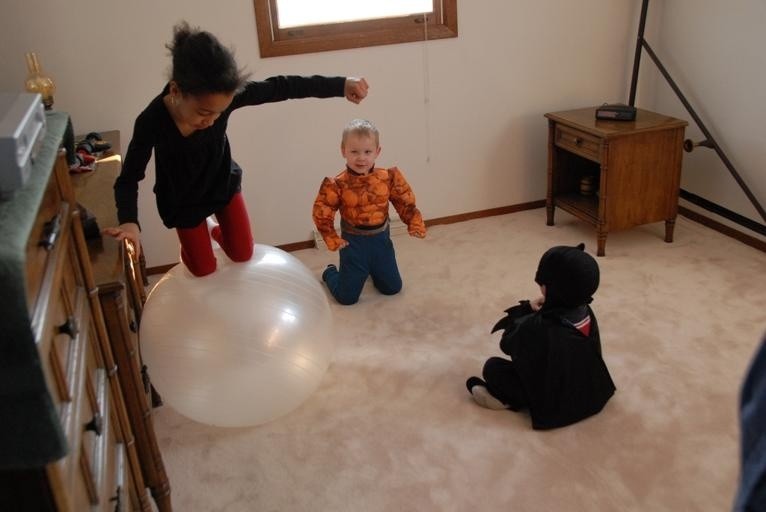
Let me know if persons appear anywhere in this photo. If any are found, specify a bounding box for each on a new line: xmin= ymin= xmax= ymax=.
xmin=99 ymin=20 xmax=371 ymax=276
xmin=464 ymin=244 xmax=617 ymax=431
xmin=730 ymin=331 xmax=766 ymax=512
xmin=312 ymin=118 xmax=426 ymax=306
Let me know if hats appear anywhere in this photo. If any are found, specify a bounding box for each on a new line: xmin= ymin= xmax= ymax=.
xmin=534 ymin=244 xmax=599 ymax=312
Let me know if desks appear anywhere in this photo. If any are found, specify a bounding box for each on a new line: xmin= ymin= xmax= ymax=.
xmin=544 ymin=102 xmax=689 ymax=257
xmin=74 ymin=130 xmax=173 ymax=511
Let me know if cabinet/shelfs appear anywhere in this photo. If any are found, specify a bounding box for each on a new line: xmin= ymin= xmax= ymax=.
xmin=0 ymin=110 xmax=175 ymax=511
